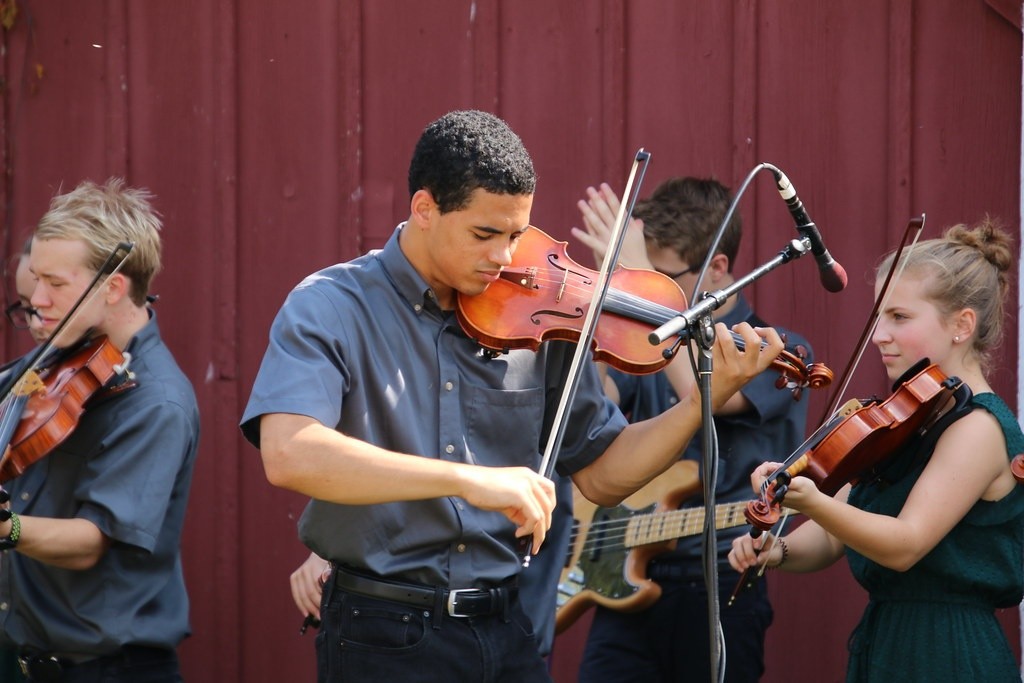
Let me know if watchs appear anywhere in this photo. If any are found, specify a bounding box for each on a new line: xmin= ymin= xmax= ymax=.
xmin=1 ymin=512 xmax=21 ymax=550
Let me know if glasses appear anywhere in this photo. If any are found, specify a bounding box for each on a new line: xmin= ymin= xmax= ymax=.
xmin=6 ymin=301 xmax=40 ymax=330
xmin=654 ymin=256 xmax=714 ymax=281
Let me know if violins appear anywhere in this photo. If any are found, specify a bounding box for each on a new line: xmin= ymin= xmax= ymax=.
xmin=456 ymin=223 xmax=834 ymax=402
xmin=745 ymin=357 xmax=972 ymax=537
xmin=1 ymin=333 xmax=131 ymax=519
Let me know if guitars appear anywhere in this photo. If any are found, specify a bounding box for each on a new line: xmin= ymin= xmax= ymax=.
xmin=554 ymin=458 xmax=800 ymax=633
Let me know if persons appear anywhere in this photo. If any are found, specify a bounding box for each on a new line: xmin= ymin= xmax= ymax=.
xmin=240 ymin=112 xmax=815 ymax=682
xmin=0 ymin=180 xmax=203 ymax=683
xmin=727 ymin=220 xmax=1024 ymax=683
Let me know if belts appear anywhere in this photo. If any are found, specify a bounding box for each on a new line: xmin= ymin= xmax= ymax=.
xmin=336 ymin=572 xmax=517 ymax=618
xmin=16 ymin=652 xmax=88 ymax=683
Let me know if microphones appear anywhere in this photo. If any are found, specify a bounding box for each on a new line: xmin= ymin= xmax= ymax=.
xmin=775 ymin=169 xmax=847 ymax=293
